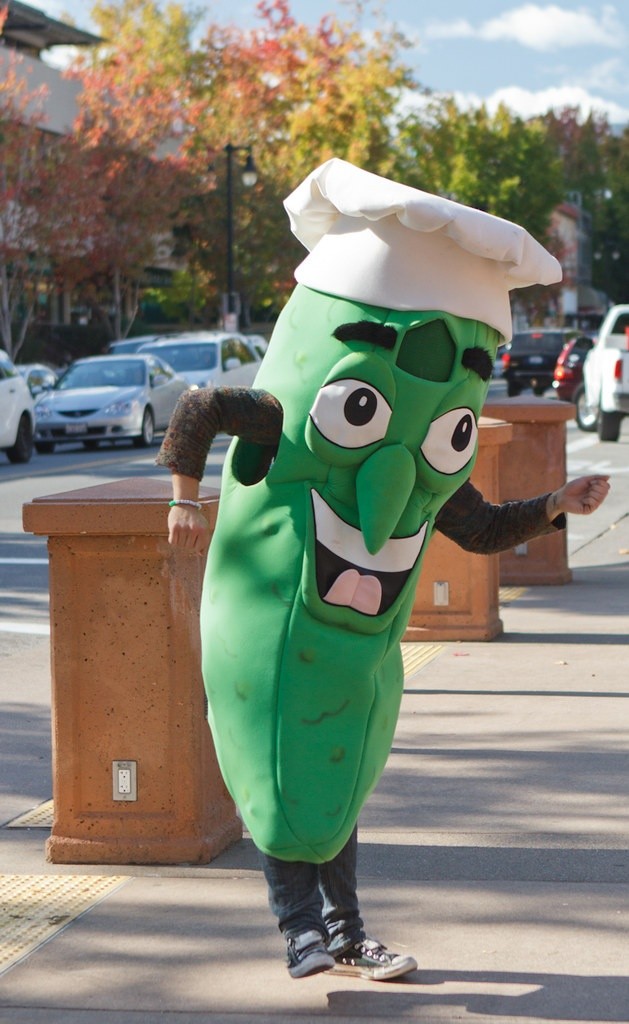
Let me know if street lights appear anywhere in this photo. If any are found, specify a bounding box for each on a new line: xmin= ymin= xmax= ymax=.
xmin=223 ymin=142 xmax=259 ymax=332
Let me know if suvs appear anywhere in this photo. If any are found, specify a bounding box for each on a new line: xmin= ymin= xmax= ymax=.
xmin=134 ymin=331 xmax=263 ymax=392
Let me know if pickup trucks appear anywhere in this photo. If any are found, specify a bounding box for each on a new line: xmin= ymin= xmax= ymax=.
xmin=583 ymin=304 xmax=629 ymax=444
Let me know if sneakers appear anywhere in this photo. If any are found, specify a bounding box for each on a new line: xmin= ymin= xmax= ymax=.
xmin=285 ymin=923 xmax=336 ymax=978
xmin=323 ymin=938 xmax=417 ymax=980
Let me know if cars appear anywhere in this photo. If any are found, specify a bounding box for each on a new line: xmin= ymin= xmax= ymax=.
xmin=552 ymin=336 xmax=594 ymax=401
xmin=15 ymin=364 xmax=58 ymax=399
xmin=34 ymin=353 xmax=192 ymax=455
xmin=106 ymin=333 xmax=167 ymax=355
xmin=500 ymin=329 xmax=585 ymax=400
xmin=0 ymin=351 xmax=37 ymax=464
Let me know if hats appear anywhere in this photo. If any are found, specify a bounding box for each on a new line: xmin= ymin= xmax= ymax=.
xmin=281 ymin=158 xmax=563 ymax=346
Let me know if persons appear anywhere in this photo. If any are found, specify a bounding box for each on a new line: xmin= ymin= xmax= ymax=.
xmin=153 ymin=156 xmax=611 ymax=979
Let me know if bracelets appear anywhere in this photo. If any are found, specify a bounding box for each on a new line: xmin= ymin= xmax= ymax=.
xmin=168 ymin=500 xmax=203 ymax=510
